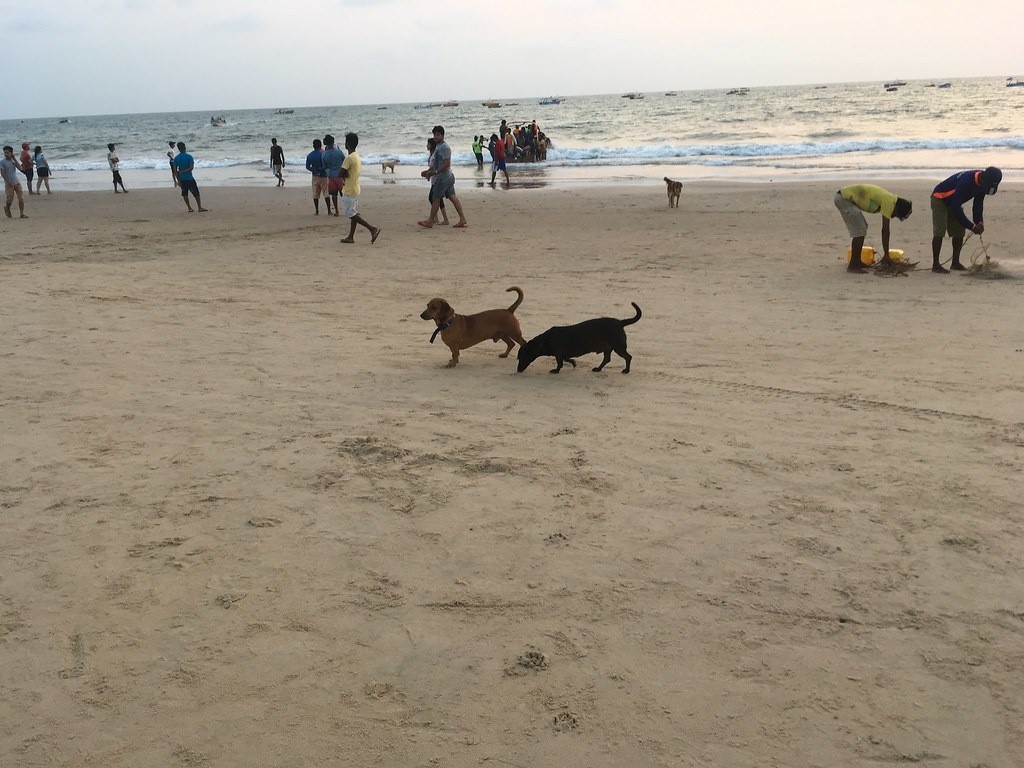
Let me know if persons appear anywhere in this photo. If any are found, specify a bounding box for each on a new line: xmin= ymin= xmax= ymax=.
xmin=417 ymin=126 xmax=467 ymax=228
xmin=472 ymin=135 xmax=489 ymax=164
xmin=834 ymin=184 xmax=913 ymax=273
xmin=0 ymin=146 xmax=30 ymax=218
xmin=270 ymin=138 xmax=285 ymax=187
xmin=108 ymin=143 xmax=129 ymax=193
xmin=20 ymin=143 xmax=36 ymax=195
xmin=929 ymin=166 xmax=1002 ymax=274
xmin=167 ymin=141 xmax=180 ymax=187
xmin=499 ymin=119 xmax=550 ymax=163
xmin=487 ymin=134 xmax=510 ymax=185
xmin=173 ymin=142 xmax=208 ymax=212
xmin=338 ymin=132 xmax=381 ymax=243
xmin=28 ymin=146 xmax=55 ymax=195
xmin=305 ymin=135 xmax=346 ymax=217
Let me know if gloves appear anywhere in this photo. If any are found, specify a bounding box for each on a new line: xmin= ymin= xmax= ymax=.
xmin=971 ymin=221 xmax=984 ymax=234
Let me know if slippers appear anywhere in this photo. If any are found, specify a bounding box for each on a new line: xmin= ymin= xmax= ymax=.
xmin=418 ymin=220 xmax=433 ymax=227
xmin=199 ymin=208 xmax=207 ymax=212
xmin=370 ymin=227 xmax=381 ymax=244
xmin=453 ymin=223 xmax=468 ymax=227
xmin=341 ymin=239 xmax=354 ymax=243
xmin=4 ymin=206 xmax=12 ymax=218
xmin=188 ymin=209 xmax=194 ymax=212
xmin=18 ymin=214 xmax=29 ymax=218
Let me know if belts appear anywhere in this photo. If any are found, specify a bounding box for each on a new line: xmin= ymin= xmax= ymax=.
xmin=837 ymin=190 xmax=841 ymax=194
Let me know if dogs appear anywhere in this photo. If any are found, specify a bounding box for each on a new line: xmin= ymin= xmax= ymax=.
xmin=420 ymin=286 xmax=528 ymax=369
xmin=515 ymin=302 xmax=642 ymax=375
xmin=382 ymin=159 xmax=400 ymax=174
xmin=664 ymin=177 xmax=683 ymax=208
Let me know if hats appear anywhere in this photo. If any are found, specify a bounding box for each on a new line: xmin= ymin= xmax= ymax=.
xmin=22 ymin=143 xmax=30 ymax=149
xmin=323 ymin=134 xmax=334 ymax=144
xmin=979 ymin=167 xmax=1002 ymax=196
xmin=169 ymin=141 xmax=176 ymax=146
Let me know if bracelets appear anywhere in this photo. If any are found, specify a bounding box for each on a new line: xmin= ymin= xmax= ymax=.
xmin=436 ymin=170 xmax=438 ymax=174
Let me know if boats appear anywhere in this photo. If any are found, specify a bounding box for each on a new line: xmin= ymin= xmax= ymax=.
xmin=629 ymin=96 xmax=645 ymax=99
xmin=938 ymin=82 xmax=951 ymax=88
xmin=210 ymin=116 xmax=226 ymax=126
xmin=1005 ymin=77 xmax=1024 ymax=87
xmin=539 ymin=96 xmax=566 ymax=105
xmin=886 ymin=87 xmax=897 ymax=92
xmin=412 ymin=98 xmax=520 ymax=110
xmin=275 ymin=109 xmax=295 ymax=114
xmin=884 ymin=82 xmax=906 ymax=87
xmin=376 ymin=106 xmax=388 ymax=110
xmin=925 ymin=84 xmax=936 ymax=88
xmin=726 ymin=87 xmax=751 ymax=95
xmin=58 ymin=119 xmax=70 ymax=123
xmin=814 ymin=85 xmax=827 ymax=90
xmin=622 ymin=93 xmax=635 ymax=98
xmin=665 ymin=92 xmax=678 ymax=96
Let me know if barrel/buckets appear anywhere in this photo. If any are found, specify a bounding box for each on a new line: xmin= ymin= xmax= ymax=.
xmin=889 ymin=249 xmax=904 ymax=262
xmin=847 ymin=246 xmax=875 ymax=265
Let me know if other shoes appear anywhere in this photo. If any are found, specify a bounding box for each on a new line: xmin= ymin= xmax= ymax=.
xmin=334 ymin=209 xmax=340 ymax=216
xmin=847 ymin=268 xmax=869 ymax=273
xmin=932 ymin=265 xmax=950 ymax=273
xmin=438 ymin=222 xmax=449 ymax=225
xmin=950 ymin=264 xmax=965 ymax=270
